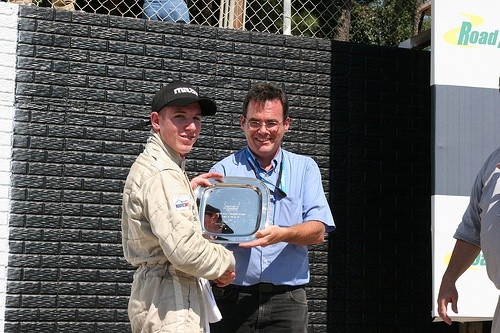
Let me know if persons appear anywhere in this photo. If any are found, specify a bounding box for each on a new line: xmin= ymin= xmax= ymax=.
xmin=196 ymin=198 xmax=234 ymax=233
xmin=437 ymin=145 xmax=500 ymax=333
xmin=143 ymin=0 xmax=190 ymax=24
xmin=192 ymin=84 xmax=337 ymax=333
xmin=120 ymin=82 xmax=236 ymax=332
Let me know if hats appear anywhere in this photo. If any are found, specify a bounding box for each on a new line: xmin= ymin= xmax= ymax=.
xmin=205 ymin=204 xmax=234 ymax=233
xmin=150 ymin=80 xmax=217 ymax=116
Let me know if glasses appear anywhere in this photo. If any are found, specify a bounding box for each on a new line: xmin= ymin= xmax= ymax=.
xmin=244 ymin=118 xmax=286 ymax=130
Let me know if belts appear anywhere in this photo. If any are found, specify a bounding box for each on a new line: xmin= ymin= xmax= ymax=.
xmin=226 ymin=282 xmax=305 ymax=294
